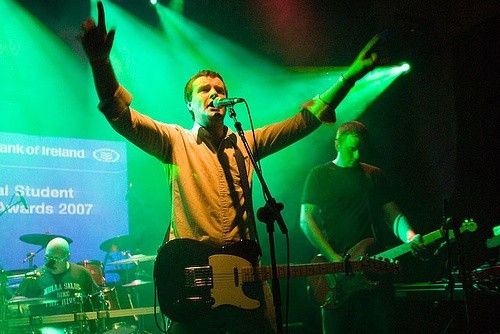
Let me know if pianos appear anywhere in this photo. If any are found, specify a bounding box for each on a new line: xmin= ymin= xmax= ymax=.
xmin=0 ymin=306 xmax=162 ymax=334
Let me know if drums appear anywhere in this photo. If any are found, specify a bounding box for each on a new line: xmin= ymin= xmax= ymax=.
xmin=82 ymin=286 xmax=123 ymax=334
xmin=96 ymin=321 xmax=152 ymax=333
xmin=76 ymin=259 xmax=107 ymax=287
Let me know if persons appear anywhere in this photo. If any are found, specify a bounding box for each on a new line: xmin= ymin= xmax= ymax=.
xmin=14 ymin=237 xmax=98 ymax=334
xmin=81 ymin=0 xmax=382 ymax=334
xmin=300 ymin=122 xmax=426 ymax=334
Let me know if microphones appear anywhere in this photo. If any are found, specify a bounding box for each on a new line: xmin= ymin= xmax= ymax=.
xmin=212 ymin=96 xmax=246 ymax=108
xmin=23 ymin=269 xmax=42 ymax=278
xmin=19 ymin=192 xmax=28 ymax=209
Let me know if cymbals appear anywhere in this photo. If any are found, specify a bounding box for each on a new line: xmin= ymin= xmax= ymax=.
xmin=105 ymin=254 xmax=158 ymax=266
xmin=6 ymin=297 xmax=46 ymax=305
xmin=19 ymin=233 xmax=73 ymax=246
xmin=99 ymin=235 xmax=144 ymax=252
xmin=2 ymin=269 xmax=37 ymax=277
xmin=121 ymin=279 xmax=153 ymax=287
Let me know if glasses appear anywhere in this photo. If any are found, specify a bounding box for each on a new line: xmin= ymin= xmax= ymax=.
xmin=44 ymin=255 xmax=67 ymax=262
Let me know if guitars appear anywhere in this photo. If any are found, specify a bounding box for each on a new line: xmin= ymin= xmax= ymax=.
xmin=305 ymin=218 xmax=480 ymax=309
xmin=153 ymin=239 xmax=408 ymax=320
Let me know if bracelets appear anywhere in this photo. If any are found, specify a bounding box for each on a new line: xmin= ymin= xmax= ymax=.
xmin=340 ymin=76 xmax=354 ymax=87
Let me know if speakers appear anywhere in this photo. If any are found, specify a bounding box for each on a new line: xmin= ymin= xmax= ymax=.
xmin=392 ymin=286 xmax=471 ymax=333
